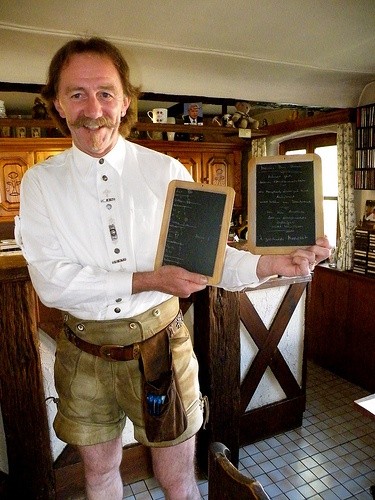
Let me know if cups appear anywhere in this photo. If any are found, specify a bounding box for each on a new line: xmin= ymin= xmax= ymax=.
xmin=16 ymin=127 xmax=26 ymax=138
xmin=1 ymin=127 xmax=10 ymax=136
xmin=147 ymin=131 xmax=162 ymax=140
xmin=167 ymin=132 xmax=175 ymax=141
xmin=328 ymin=246 xmax=338 ymax=268
xmin=31 ymin=127 xmax=41 ymax=138
xmin=147 ymin=108 xmax=168 ymax=123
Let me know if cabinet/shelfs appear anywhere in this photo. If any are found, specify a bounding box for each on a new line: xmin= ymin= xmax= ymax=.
xmin=309 ymin=259 xmax=375 ymax=390
xmin=0 ymin=118 xmax=273 ymax=257
xmin=354 ymin=103 xmax=375 ymax=190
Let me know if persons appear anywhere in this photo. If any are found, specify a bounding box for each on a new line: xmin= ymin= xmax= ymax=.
xmin=364 ymin=200 xmax=375 ymax=221
xmin=19 ymin=38 xmax=333 ymax=500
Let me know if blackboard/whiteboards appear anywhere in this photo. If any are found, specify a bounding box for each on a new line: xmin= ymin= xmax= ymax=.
xmin=248 ymin=154 xmax=323 ymax=255
xmin=155 ymin=179 xmax=235 ymax=290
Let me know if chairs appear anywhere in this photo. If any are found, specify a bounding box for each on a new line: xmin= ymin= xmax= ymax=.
xmin=207 ymin=441 xmax=272 ymax=500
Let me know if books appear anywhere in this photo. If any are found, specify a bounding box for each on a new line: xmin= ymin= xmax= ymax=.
xmin=351 ymin=109 xmax=375 ymax=277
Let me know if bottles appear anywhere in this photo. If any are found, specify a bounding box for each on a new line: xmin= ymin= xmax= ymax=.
xmin=236 ymin=214 xmax=248 ymax=241
xmin=263 ymin=119 xmax=267 ymax=126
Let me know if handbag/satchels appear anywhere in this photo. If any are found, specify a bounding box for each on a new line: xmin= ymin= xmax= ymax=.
xmin=138 ymin=329 xmax=187 ymax=443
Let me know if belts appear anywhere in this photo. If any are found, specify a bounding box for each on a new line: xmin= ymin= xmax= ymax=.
xmin=64 ymin=309 xmax=183 ymax=361
